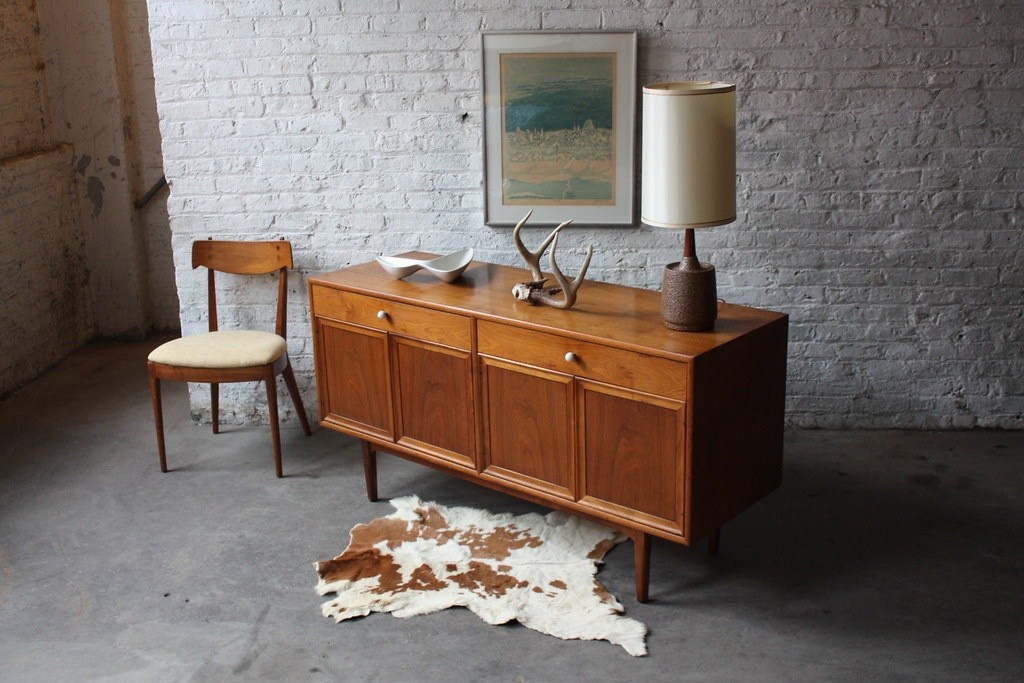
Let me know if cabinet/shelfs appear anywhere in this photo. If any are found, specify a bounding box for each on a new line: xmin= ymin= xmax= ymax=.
xmin=309 ymin=252 xmax=555 ymax=502
xmin=477 ymin=280 xmax=792 ymax=604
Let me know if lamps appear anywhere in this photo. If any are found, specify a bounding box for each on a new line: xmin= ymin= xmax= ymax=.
xmin=641 ymin=81 xmax=738 ymax=334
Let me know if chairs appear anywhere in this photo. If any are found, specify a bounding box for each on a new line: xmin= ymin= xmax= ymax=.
xmin=146 ymin=241 xmax=313 ymax=478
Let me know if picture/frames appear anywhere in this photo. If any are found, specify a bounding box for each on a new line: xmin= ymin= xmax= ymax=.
xmin=479 ymin=28 xmax=638 ymax=228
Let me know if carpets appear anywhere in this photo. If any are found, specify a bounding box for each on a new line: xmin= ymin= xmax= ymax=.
xmin=315 ymin=492 xmax=650 ymax=657
